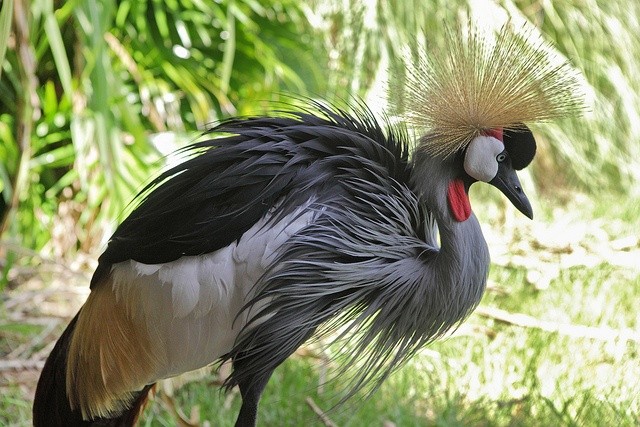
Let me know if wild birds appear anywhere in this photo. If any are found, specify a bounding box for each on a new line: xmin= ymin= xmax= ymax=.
xmin=32 ymin=5 xmax=595 ymax=427
